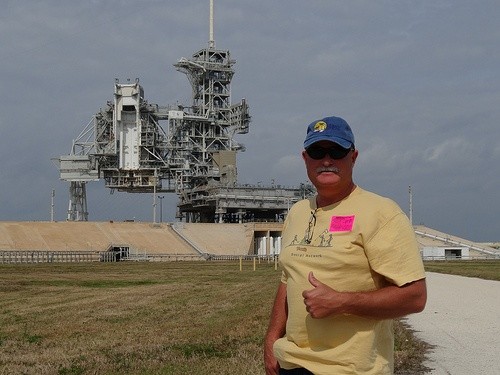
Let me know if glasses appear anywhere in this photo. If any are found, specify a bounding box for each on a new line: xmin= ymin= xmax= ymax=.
xmin=306 ymin=146 xmax=355 ymax=160
xmin=304 ymin=210 xmax=316 ymax=243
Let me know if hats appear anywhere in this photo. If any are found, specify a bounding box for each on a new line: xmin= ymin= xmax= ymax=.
xmin=303 ymin=117 xmax=354 ymax=149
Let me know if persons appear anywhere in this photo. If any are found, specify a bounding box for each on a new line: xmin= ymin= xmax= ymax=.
xmin=262 ymin=116 xmax=428 ymax=375
